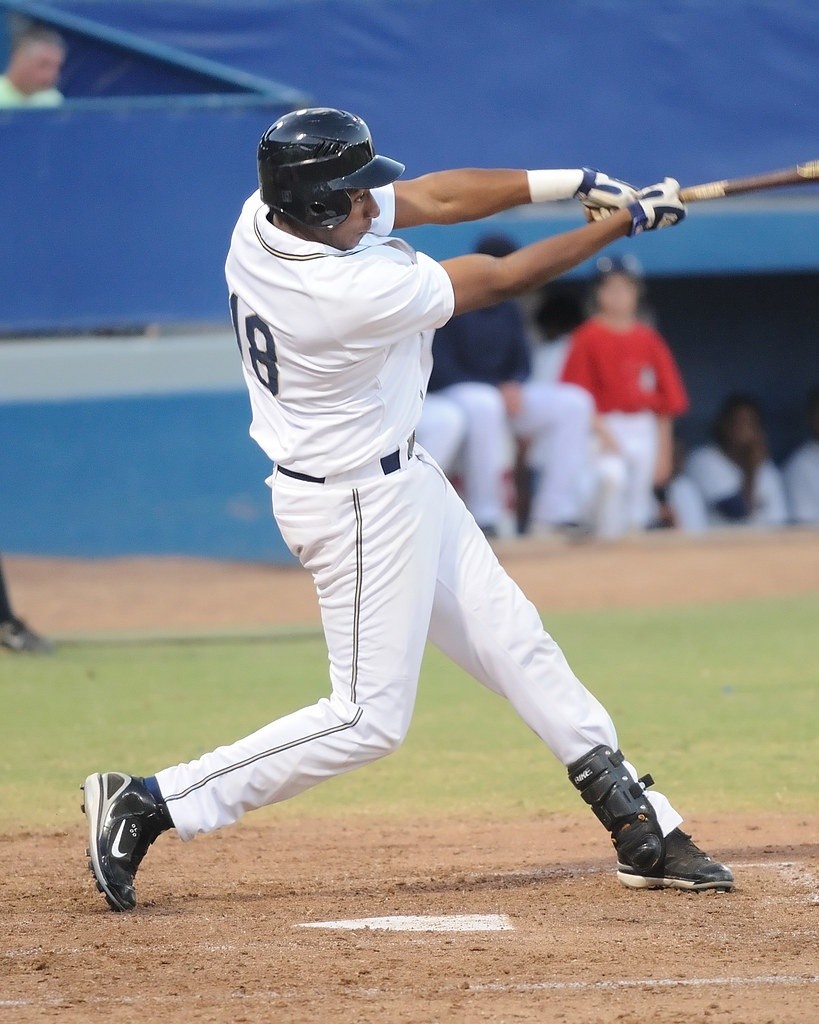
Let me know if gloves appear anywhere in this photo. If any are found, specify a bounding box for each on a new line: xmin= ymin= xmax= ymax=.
xmin=573 ymin=167 xmax=642 ymax=223
xmin=627 ymin=176 xmax=688 ymax=237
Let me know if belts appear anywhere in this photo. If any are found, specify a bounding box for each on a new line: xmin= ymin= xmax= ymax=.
xmin=277 ymin=431 xmax=415 ymax=483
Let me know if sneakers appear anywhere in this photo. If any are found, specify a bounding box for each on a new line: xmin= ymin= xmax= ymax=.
xmin=79 ymin=772 xmax=176 ymax=911
xmin=616 ymin=828 xmax=734 ymax=890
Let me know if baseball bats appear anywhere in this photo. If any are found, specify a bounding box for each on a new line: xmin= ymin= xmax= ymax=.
xmin=585 ymin=160 xmax=819 ymax=228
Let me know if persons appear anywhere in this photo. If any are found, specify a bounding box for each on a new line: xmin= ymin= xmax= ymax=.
xmin=414 ymin=235 xmax=819 ymax=537
xmin=0 ymin=26 xmax=67 ymax=108
xmin=81 ymin=109 xmax=734 ymax=913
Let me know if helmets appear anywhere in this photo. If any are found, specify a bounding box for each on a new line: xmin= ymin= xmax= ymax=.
xmin=257 ymin=106 xmax=404 ymax=229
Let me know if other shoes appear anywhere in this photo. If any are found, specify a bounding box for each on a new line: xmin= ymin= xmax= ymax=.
xmin=0 ymin=619 xmax=52 ymax=654
